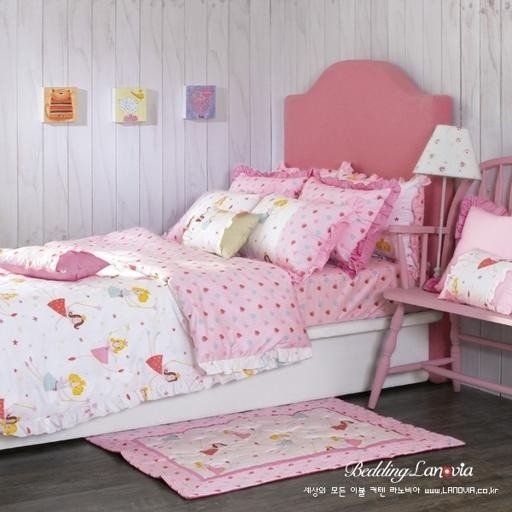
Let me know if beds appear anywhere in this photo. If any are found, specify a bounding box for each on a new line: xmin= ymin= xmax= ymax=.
xmin=0 ymin=59 xmax=452 ymax=449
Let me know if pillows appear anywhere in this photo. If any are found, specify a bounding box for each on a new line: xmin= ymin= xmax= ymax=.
xmin=1 ymin=246 xmax=110 ymax=281
xmin=421 ymin=195 xmax=512 ymax=316
xmin=165 ymin=161 xmax=431 ymax=283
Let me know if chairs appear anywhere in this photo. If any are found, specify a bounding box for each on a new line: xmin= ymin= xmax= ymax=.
xmin=366 ymin=155 xmax=512 ymax=412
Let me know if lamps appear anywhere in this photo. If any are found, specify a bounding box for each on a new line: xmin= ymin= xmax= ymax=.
xmin=411 ymin=123 xmax=482 ymax=293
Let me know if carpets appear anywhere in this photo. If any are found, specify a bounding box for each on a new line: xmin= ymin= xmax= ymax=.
xmin=85 ymin=398 xmax=465 ymax=500
